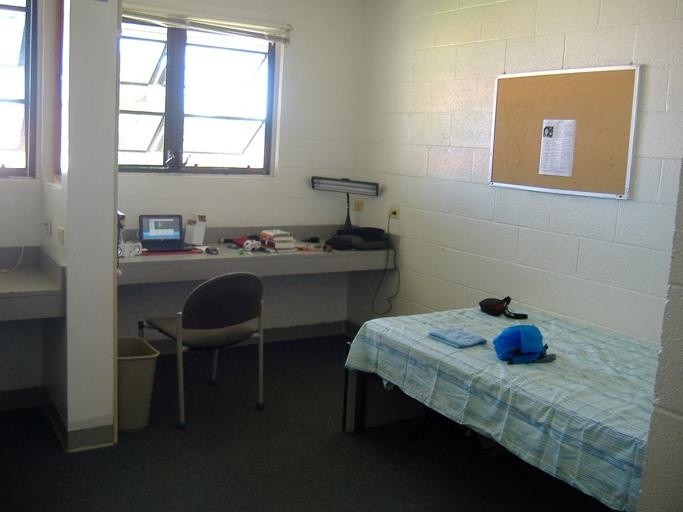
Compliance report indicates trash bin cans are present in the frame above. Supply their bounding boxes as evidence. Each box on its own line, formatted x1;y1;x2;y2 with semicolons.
117;335;160;431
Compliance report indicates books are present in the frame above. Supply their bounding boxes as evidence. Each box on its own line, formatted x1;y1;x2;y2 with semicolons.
258;229;302;256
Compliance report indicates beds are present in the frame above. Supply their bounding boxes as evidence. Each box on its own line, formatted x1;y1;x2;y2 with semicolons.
341;300;660;512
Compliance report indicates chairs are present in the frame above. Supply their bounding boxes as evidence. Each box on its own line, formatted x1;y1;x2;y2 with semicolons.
136;271;265;432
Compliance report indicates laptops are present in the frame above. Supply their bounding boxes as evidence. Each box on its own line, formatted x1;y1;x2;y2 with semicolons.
138;214;193;252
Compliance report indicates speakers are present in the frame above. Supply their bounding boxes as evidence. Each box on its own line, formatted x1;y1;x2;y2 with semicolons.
118;242;142;258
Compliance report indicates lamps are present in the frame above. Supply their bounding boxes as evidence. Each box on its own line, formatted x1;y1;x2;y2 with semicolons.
310;175;378;235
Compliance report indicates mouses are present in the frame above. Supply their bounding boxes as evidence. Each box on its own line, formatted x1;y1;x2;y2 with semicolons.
205;246;219;255
